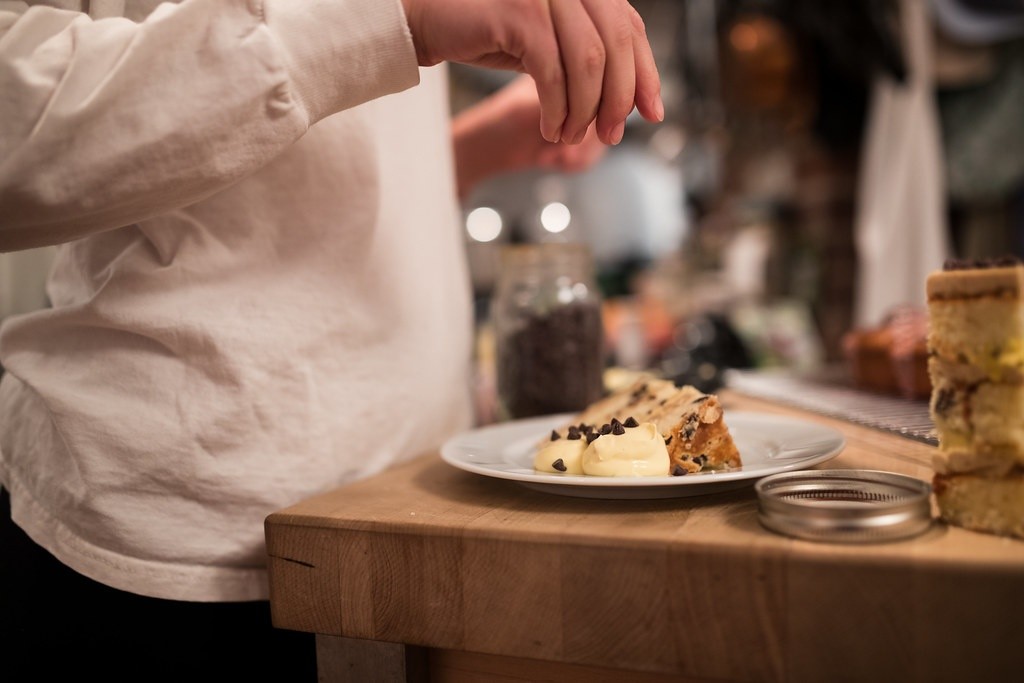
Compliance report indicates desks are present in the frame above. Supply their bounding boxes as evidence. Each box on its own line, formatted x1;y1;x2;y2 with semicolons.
263;365;1024;683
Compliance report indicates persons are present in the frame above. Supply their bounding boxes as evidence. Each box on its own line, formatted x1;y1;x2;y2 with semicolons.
0;0;666;683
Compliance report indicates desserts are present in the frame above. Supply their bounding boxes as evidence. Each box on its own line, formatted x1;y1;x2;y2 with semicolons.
925;255;1024;541
534;376;743;478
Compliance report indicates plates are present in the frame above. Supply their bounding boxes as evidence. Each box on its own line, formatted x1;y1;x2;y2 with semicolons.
439;412;847;501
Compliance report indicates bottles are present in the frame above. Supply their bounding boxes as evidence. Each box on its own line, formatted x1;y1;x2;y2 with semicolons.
488;243;604;421
